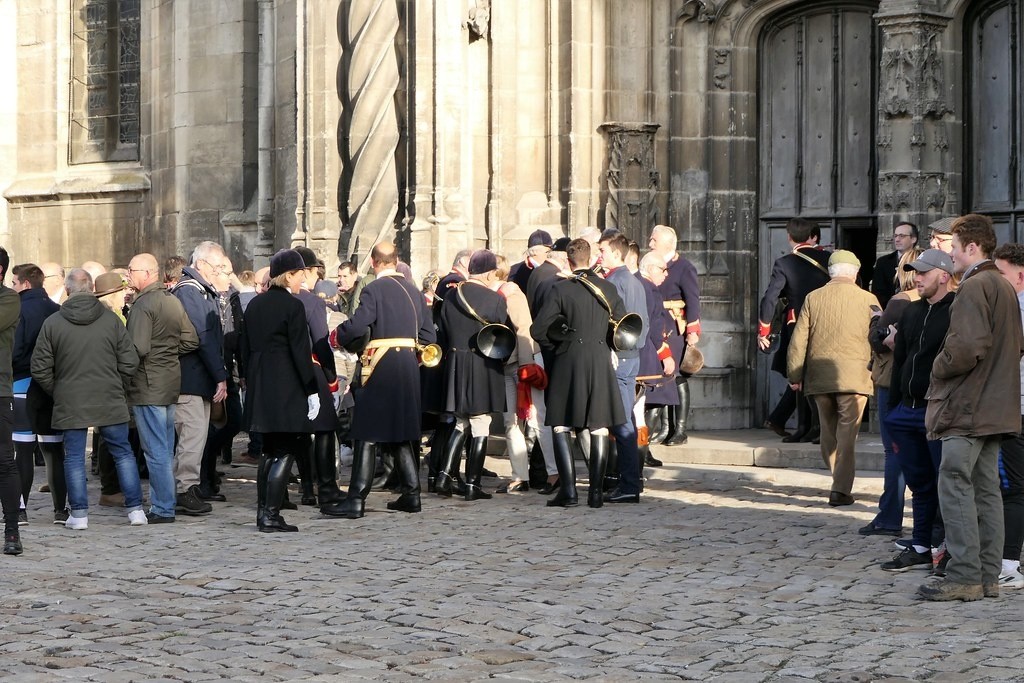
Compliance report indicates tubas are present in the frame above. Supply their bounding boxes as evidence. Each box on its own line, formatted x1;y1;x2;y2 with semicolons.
609;313;644;352
477;323;519;360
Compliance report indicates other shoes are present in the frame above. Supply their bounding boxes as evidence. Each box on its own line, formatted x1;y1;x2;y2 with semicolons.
831;489;1024;602
0;430;255;555
764;417;820;445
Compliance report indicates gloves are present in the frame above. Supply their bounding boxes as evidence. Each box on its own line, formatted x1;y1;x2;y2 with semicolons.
331;390;340;410
307;393;320;419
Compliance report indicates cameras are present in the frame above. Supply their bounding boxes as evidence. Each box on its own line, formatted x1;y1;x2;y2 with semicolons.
876;327;891;341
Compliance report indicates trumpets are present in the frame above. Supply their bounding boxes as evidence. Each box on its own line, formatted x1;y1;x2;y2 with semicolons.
413;341;442;368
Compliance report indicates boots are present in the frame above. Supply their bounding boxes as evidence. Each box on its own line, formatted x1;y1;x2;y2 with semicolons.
256;381;691;533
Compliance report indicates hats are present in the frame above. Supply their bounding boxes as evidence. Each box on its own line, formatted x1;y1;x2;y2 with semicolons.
315;279;337;301
270;248;310;278
396;262;412;281
468;250;500;274
929;215;960;233
830;249;861;268
901;247;956;277
292;246;323;267
94;272;128;297
529;229;552;248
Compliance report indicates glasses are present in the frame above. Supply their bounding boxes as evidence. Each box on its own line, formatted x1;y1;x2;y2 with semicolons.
892;231;909;238
203;260;225;273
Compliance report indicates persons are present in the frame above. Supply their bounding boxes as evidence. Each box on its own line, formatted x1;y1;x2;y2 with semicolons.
860;217;964;576
993;243;1024;588
787;250;883;505
30;267;149;528
579;225;699;503
231;246;376;532
529;238;625;507
490;230;572;496
434;251;507;501
94;241;244;522
757;220;833;442
372;251;465;494
0;247;104;553
320;243;437;517
919;214;1024;601
